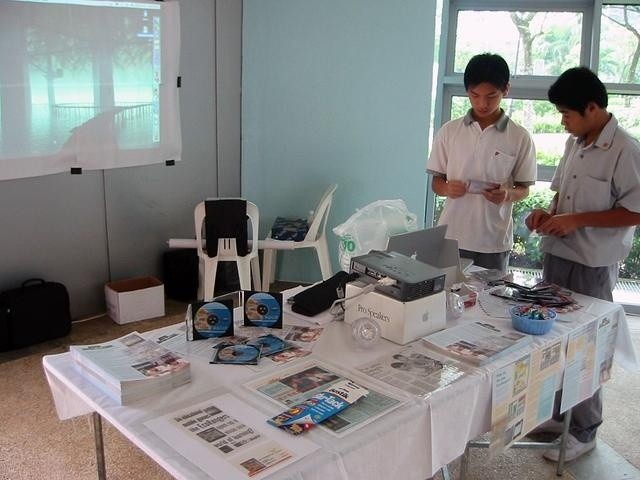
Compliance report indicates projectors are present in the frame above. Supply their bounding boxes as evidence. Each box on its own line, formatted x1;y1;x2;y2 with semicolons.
349;249;447;303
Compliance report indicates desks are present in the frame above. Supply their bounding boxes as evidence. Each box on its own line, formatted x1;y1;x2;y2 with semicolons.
43;264;624;480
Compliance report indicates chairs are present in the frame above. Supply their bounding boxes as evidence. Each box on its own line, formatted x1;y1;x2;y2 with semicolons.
195;184;342;302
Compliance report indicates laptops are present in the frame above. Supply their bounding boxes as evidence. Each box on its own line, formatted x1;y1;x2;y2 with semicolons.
438;239;474;272
385;224;448;266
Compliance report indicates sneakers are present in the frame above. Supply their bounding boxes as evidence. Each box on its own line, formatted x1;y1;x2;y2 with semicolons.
543;432;596;463
530;418;565;434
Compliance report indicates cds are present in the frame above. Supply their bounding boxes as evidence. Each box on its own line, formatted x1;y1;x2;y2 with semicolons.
194;301;232;338
248;336;285;353
244;293;281;328
218;344;260;362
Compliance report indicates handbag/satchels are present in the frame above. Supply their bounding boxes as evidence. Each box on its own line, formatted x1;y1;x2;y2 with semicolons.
1;279;71;353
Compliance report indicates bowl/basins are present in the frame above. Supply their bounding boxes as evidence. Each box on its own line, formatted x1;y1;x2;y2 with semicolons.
352;317;380;348
447;292;465;320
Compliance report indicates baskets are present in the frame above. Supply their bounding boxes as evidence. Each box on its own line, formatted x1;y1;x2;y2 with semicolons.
509;304;558;335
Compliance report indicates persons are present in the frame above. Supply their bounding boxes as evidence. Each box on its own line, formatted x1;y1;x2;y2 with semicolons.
525;64;640;465
144;365;169;378
270;327;333;393
164;357;188;372
425;50;538;271
393;354;443;373
391;362;431;378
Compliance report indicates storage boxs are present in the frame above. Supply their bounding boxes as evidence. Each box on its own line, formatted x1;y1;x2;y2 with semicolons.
104;274;165;326
